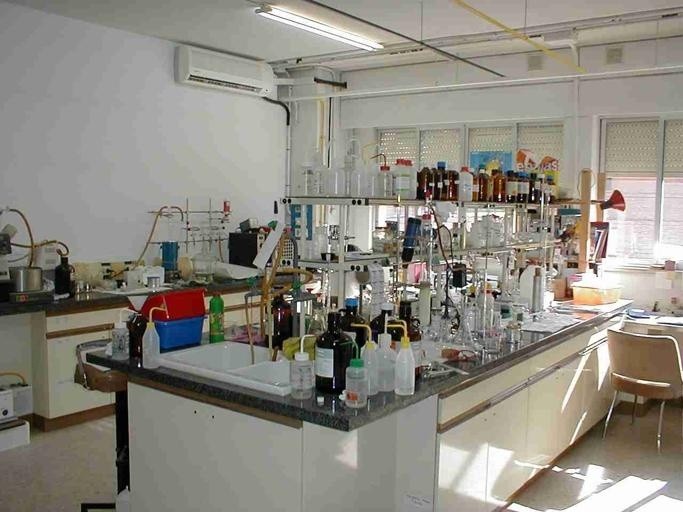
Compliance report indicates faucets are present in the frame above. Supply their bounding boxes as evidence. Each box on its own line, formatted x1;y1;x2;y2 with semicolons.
242;274;260;300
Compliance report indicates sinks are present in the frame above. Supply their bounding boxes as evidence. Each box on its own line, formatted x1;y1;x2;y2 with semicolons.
160;339;272;373
232;355;293;398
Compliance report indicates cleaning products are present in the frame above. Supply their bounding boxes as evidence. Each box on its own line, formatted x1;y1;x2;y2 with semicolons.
209;291;224;341
393;159;557;201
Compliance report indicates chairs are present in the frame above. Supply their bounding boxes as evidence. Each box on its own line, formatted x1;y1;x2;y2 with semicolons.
601;327;682;456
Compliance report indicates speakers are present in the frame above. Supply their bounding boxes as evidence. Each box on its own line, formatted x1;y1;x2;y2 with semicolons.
229;232;271;270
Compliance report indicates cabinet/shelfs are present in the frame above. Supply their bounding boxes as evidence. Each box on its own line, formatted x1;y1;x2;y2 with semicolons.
30;282;262;431
283;193;562;340
431;312;626;511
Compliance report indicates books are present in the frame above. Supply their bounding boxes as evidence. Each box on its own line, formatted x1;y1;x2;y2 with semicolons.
656;314;682;326
627;307;649;318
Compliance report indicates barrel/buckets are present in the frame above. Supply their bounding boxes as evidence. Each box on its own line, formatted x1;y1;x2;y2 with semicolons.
520;259;546;309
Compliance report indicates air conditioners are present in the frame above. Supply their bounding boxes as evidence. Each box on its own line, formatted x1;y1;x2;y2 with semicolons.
173;45;271;99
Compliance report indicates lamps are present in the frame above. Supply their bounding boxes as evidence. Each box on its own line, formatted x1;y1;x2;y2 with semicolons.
255;5;384;52
591;189;625;212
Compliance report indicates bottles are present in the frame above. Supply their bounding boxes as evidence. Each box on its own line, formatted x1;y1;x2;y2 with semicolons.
108;304;171;370
208;290;226;344
264;153;562;414
52;257;76;298
